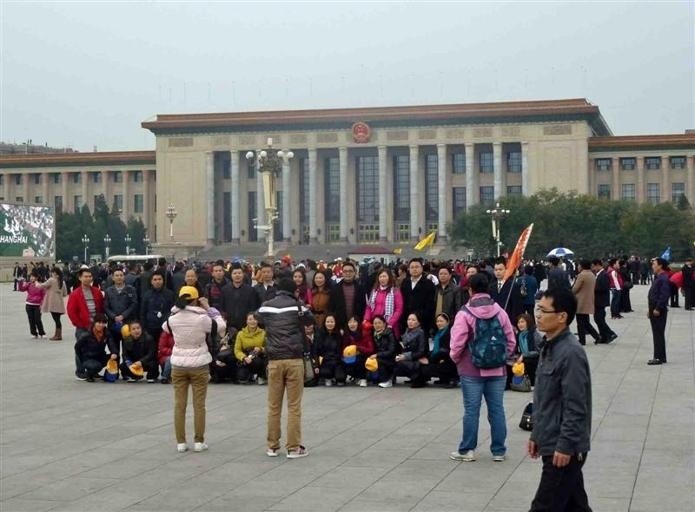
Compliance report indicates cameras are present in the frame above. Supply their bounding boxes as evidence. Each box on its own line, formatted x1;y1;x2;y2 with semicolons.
17;277;24;282
198;298;200;306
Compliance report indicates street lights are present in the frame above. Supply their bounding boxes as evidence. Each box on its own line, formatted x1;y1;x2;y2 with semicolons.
245;138;294;256
143;235;150;255
124;233;132;256
82;234;90;263
166;204;177;242
103;234;111;258
486;203;511;257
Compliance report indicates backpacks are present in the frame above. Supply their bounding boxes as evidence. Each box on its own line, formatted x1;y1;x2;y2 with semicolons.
460;305;507;368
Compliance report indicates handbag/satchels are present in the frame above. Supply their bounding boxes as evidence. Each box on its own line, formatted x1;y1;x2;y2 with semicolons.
520;284;528;297
624;280;632;289
509;374;531;391
520;404;533;431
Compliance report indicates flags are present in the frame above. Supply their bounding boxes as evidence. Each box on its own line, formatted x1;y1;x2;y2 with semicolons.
414;232;435;251
660;246;673;263
502;222;535;282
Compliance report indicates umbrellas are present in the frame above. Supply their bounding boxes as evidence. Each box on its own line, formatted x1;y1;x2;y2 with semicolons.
545;246;575;259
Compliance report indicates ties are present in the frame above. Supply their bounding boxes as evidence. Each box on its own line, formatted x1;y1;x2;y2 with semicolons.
498;282;502;293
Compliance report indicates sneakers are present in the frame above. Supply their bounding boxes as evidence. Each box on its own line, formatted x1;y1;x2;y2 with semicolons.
178;442;188;451
75;369;103;382
31;334;47;338
267;448;280;457
306;377;463;388
492;455;505;461
595;333;617;343
194;442;209;452
648;358;666;365
239;373;267;385
127;378;169;384
449;449;476;462
288;446;309;458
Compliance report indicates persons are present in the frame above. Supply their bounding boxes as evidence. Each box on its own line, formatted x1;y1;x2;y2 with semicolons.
13;255;695;391
161;286;226;453
527;287;592;512
253;277;315;458
449;273;516;461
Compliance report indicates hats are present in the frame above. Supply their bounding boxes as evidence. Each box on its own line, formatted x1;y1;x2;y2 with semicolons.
125;359;145;376
342;345;358;363
513;363;524;380
179;286;199;300
105;358;118;382
279;256;290;265
366;356;380;379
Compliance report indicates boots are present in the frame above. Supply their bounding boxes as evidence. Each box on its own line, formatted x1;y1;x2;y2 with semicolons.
49;328;62;341
